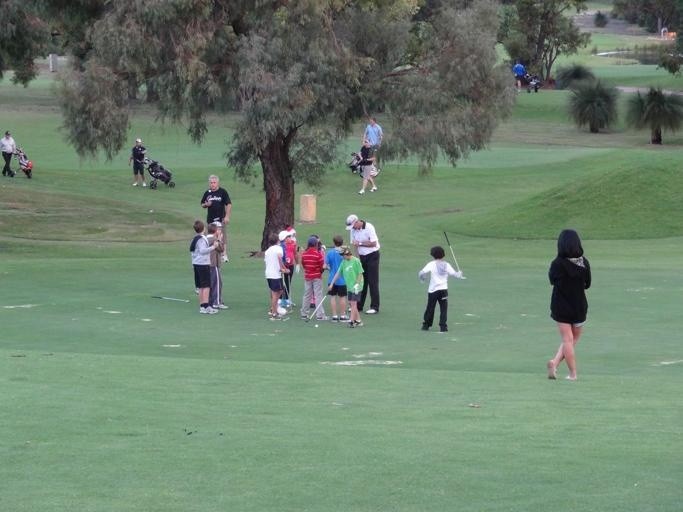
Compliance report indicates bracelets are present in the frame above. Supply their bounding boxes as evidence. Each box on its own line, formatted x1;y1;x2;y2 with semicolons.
357;242;362;246
204;204;206;207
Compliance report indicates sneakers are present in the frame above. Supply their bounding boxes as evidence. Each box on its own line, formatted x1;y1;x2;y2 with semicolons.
142;182;147;187
2;171;6;176
364;308;380;314
10;171;16;177
331;315;363;328
132;182;139;187
200;302;229;314
358;189;365;194
268;297;296;320
369;187;378;193
565;374;578;381
440;325;449;332
348;308;353;312
421;323;429;330
301;313;312;320
222;254;229;263
547;360;557;380
316;314;329;321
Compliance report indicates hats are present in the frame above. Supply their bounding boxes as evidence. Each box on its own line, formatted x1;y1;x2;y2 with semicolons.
336;246;353;255
345;214;359;231
278;225;297;242
307;237;320;245
136;139;142;143
212;221;223;227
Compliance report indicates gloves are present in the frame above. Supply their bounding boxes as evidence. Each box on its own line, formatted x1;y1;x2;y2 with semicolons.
296;263;303;273
352;284;360;295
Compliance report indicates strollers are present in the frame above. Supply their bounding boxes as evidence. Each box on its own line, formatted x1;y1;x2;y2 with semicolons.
128;154;176;189
347;141;370;177
524;74;543;94
8;148;38;180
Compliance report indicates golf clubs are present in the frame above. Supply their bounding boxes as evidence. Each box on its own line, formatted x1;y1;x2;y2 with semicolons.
444;232;467;280
305;292;330;322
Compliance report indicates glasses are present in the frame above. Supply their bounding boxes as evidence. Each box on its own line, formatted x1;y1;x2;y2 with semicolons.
8;134;11;135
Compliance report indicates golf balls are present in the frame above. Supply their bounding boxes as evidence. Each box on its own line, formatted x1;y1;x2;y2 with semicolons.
314;325;319;328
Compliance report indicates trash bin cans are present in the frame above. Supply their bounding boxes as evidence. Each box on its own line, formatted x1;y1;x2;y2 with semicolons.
300;194;317;224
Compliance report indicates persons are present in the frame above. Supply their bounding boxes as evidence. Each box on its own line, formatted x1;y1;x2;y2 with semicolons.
345;215;381;315
260;225;365;328
362;118;384;167
358;138;377;193
189;219;218;313
526;74;543;87
418;246;464;331
545;227;590;382
512;58;525;90
204;223;228;309
201;175;232;262
0;131;16;178
127;138;147;188
